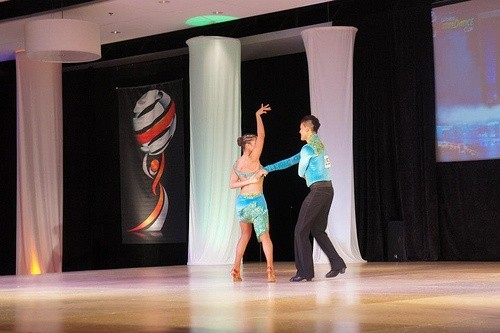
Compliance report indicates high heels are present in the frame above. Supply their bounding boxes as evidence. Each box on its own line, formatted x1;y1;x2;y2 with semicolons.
325;266;345;278
231;269;242;282
266;267;276;282
290;274;314;281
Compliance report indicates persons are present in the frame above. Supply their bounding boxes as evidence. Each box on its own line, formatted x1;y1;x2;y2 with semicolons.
229;104;276;282
254;115;346;282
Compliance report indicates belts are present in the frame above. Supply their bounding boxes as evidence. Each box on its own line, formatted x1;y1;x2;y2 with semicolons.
310;181;332;190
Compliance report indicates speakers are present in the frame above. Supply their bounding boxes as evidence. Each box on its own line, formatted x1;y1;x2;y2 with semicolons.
385;220;408;262
